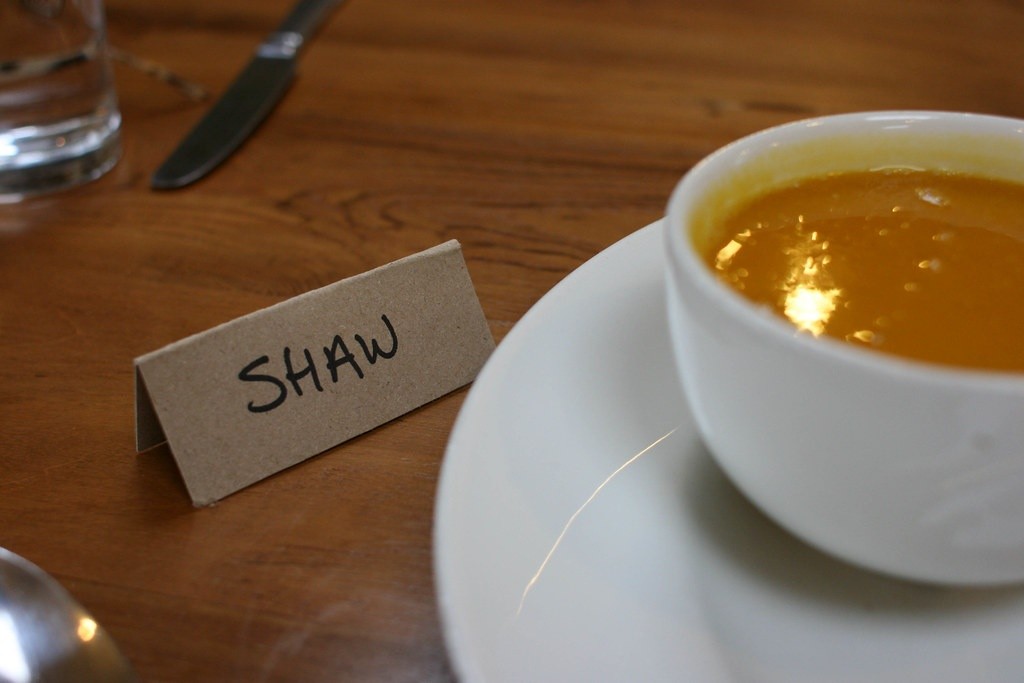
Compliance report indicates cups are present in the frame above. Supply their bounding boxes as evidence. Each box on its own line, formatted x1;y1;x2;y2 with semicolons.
1;1;123;204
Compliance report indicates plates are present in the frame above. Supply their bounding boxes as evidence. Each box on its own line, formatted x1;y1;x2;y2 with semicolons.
432;216;1023;682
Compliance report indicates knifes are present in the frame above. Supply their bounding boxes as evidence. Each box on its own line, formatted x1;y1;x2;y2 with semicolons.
150;0;340;187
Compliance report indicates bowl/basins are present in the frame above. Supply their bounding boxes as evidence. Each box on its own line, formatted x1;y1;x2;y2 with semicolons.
663;111;1024;585
